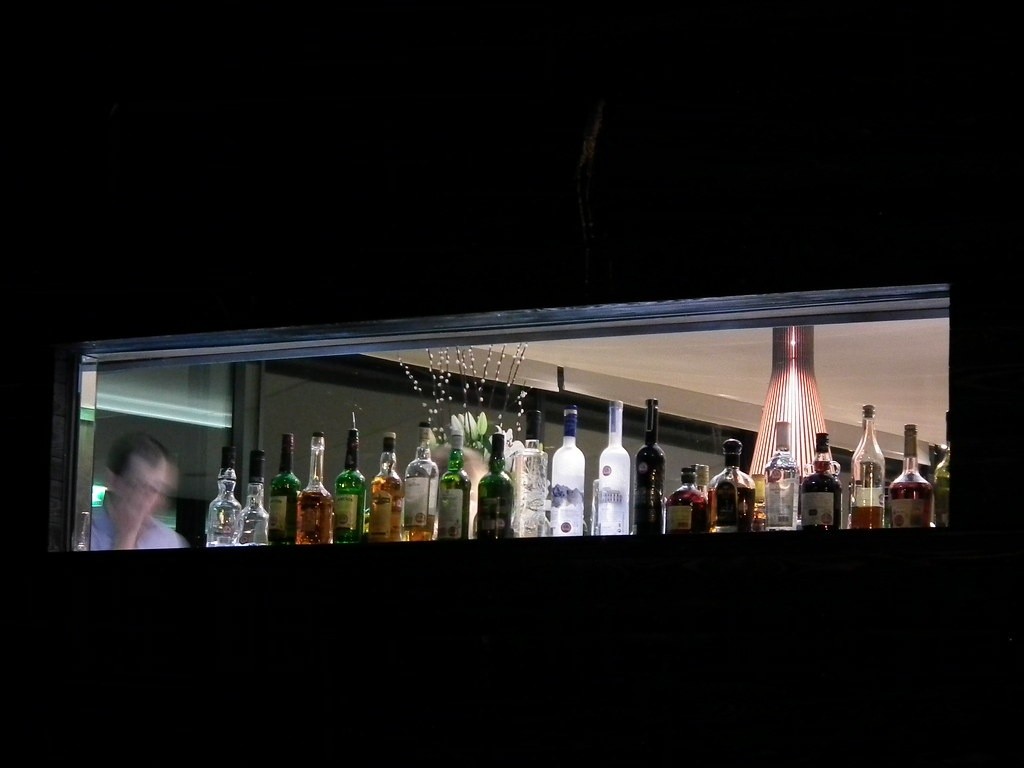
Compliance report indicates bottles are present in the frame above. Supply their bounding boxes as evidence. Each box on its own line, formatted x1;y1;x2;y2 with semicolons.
235;449;267;544
515;410;548;537
296;432;333;542
799;404;949;529
403;422;437;540
333;428;367;542
265;433;302;543
597;398;757;530
437;435;472;541
765;422;800;530
205;446;242;546
368;432;403;540
475;433;511;538
550;404;585;537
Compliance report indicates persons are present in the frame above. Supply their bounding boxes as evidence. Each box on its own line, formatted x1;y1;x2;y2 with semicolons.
92;431;191;550
429;445;491;539
